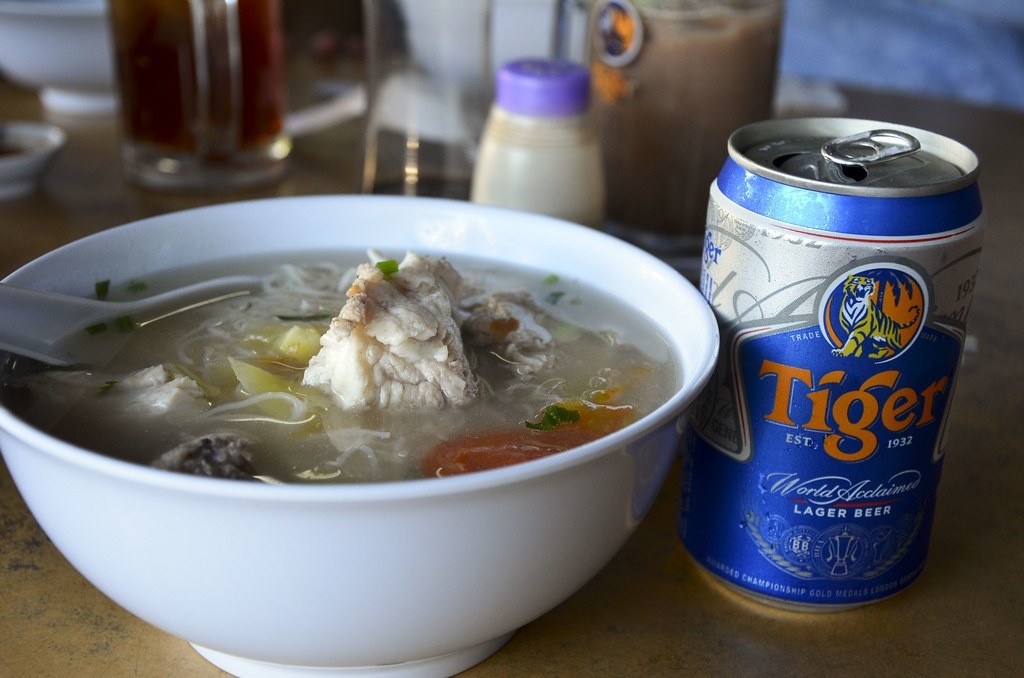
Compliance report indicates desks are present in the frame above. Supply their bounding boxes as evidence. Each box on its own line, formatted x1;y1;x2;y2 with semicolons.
0;89;1024;678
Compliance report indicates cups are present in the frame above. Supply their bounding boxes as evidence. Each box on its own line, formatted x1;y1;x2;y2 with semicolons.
579;0;785;275
362;0;493;202
101;1;292;186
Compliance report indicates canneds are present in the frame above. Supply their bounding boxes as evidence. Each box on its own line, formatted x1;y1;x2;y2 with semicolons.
678;115;985;614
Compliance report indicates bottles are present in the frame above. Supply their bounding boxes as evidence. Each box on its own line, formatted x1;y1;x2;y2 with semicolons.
466;57;607;232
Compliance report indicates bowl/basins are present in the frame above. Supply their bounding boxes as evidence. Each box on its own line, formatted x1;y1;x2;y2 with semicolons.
0;1;116;115
0;120;68;200
0;194;719;678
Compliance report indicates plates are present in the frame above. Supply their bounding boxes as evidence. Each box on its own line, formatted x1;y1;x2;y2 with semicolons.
286;80;366;138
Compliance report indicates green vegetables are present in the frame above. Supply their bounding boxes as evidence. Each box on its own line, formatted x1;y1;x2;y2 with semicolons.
41;258;581;432
